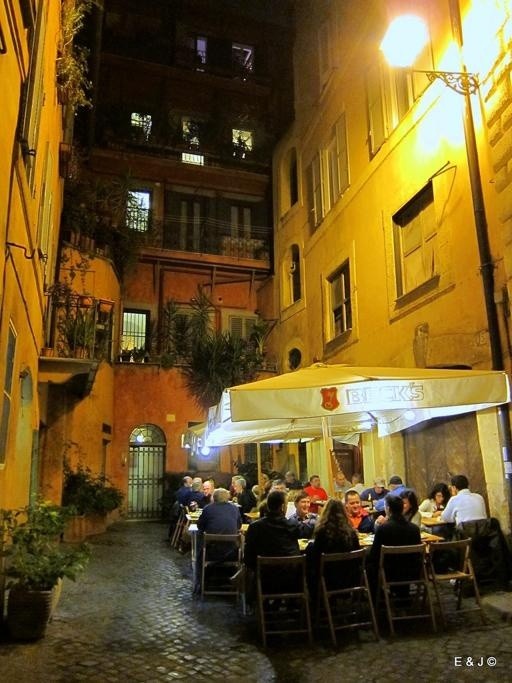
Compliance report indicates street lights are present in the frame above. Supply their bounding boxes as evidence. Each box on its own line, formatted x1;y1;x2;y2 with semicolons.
373;9;511;494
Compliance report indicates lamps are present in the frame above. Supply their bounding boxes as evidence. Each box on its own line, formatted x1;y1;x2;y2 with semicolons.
136;428;148;443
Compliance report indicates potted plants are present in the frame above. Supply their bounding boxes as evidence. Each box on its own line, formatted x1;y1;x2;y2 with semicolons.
60;455;127;544
113;295;280;371
60;163;138;257
0;495;92;640
40;284;117;364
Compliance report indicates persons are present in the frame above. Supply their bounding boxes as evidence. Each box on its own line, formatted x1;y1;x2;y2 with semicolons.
173;470;494;613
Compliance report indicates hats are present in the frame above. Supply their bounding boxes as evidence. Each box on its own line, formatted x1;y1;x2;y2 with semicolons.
388;475;401;485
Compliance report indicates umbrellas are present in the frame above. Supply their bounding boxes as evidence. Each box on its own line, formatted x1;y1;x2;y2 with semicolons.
203;357;510;497
204;403;371;488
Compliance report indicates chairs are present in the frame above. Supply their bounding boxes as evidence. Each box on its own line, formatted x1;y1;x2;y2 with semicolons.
168;495;512;654
